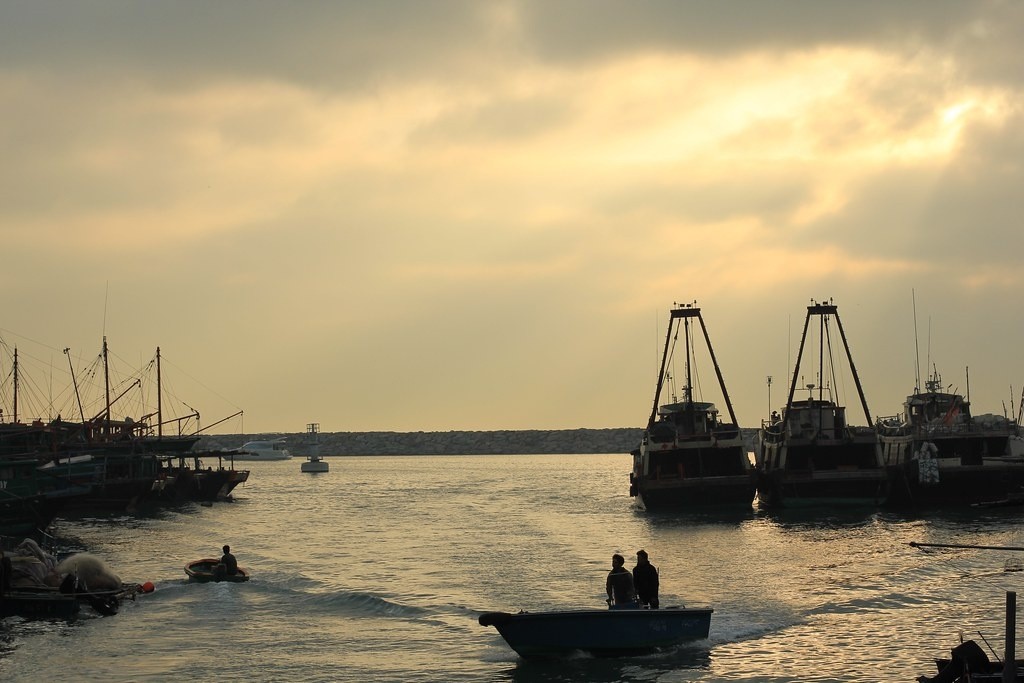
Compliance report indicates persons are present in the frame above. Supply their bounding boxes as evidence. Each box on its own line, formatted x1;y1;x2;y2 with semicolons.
633;550;659;611
218;545;238;576
606;553;634;605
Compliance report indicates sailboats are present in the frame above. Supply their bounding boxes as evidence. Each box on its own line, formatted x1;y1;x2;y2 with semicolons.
0;336;251;536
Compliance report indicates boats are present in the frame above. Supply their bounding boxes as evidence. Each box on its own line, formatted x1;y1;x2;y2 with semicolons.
478;606;714;660
755;299;894;516
223;439;293;461
184;558;249;583
877;287;1023;514
0;542;143;617
628;298;760;514
